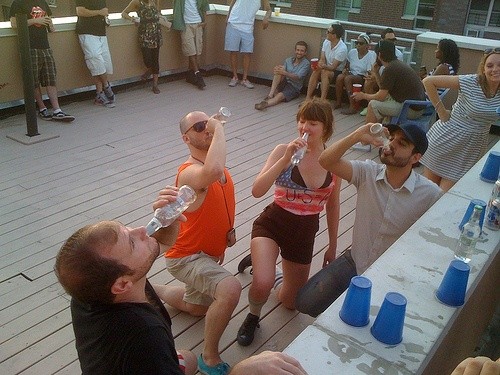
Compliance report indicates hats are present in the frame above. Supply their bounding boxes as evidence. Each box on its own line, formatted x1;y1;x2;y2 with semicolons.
385;123;428;154
359;34;370;44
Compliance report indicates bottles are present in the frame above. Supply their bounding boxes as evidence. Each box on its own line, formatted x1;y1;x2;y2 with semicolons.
214;107;231;126
454;205;483;263
291;133;309;165
370;123;390;152
145;185;197;237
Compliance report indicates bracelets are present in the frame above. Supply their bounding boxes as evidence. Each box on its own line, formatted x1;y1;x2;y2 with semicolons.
204;21;207;26
131;16;135;23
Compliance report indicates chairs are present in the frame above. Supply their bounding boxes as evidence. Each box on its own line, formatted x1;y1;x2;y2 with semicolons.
390;88;450;134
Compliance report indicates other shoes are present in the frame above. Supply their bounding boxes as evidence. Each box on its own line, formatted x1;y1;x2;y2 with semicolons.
229;79;239;86
190;72;205;87
153;87;160;94
241;79;254;88
255;101;267;110
334;104;343;110
264;95;275;101
143;71;151;81
341;109;357;114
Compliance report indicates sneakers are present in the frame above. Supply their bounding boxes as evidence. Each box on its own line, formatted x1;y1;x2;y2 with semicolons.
198;352;230;375
95;97;115;108
104;82;115;100
52;111;75;121
237;313;260;346
360;107;367;115
238;254;252;273
39;109;52;120
351;142;371;152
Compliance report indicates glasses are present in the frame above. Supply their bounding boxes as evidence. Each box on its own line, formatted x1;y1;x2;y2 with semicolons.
387;38;396;42
184;120;208;134
485;48;500;54
327;31;331;34
355;41;367;45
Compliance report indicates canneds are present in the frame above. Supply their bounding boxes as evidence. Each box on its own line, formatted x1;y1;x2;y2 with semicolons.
483;199;500;230
488;179;500;205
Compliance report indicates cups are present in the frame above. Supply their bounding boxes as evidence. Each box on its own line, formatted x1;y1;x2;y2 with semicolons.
353;84;362;95
274;8;280;16
436;260;470;306
370;292;407;345
311;59;318;69
459;199;487;235
339;276;372;327
480;151;500;183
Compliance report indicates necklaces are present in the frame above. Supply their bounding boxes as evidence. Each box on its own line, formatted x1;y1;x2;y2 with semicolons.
191;154;237;247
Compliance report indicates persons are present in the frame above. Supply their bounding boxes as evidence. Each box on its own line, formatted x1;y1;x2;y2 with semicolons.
449;356;500;375
121;0;163;94
254;41;312;110
8;0;75;122
295;122;444;318
74;0;116;108
298;22;460;152
418;48;500;194
172;0;210;88
53;220;310;375
149;111;242;375
237;96;343;347
224;0;272;89
